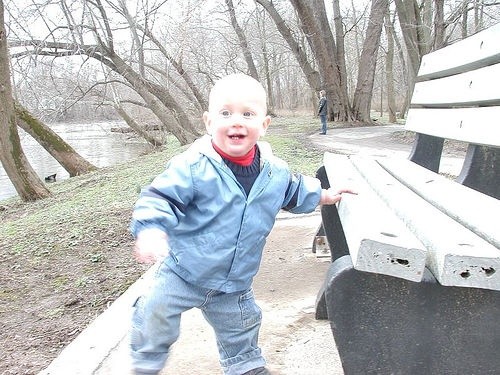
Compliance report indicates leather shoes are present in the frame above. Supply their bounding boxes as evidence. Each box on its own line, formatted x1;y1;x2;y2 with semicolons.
320;133;326;135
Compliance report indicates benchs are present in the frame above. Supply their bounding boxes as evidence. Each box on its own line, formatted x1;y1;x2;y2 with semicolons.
312;20;500;374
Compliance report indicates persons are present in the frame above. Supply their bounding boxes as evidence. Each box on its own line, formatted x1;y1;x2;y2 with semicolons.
131;74;359;375
317;89;329;135
